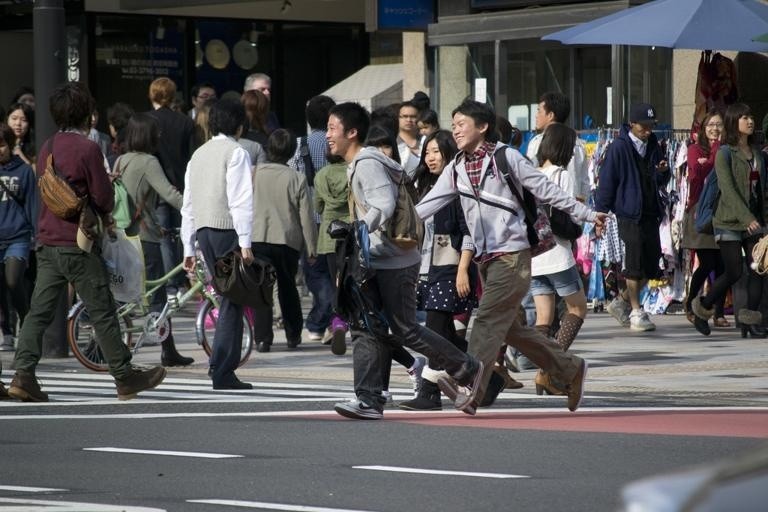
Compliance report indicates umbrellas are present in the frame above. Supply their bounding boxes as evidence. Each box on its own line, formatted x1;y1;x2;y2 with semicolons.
540;0;768;54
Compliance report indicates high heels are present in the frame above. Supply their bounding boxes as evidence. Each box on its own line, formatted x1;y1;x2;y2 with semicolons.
737;309;768;338
533;367;569;398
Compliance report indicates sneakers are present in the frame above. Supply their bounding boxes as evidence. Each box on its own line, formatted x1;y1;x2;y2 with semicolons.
437;360;484;417
257;316;350;357
606;294;657;332
334;396;383;420
491;364;523;390
568;354;588;411
410;355;426;396
380;389;394;409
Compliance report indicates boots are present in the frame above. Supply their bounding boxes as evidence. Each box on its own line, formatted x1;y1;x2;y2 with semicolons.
110;367;167;402
158;320;195;366
8;368;52;404
396;366;443;411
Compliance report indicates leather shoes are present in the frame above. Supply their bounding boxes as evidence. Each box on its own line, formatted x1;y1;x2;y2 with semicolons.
213;377;252;390
207;361;220;376
691;295;716;336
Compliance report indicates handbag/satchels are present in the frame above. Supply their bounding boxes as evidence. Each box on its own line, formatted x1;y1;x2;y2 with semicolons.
98;226;148;305
550;204;584;240
212;254;276;309
37;165;82;220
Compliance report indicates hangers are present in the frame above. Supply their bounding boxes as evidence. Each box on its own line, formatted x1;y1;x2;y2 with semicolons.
596;127;692;143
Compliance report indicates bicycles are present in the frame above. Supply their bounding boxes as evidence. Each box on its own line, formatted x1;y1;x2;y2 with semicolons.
65;227;254;375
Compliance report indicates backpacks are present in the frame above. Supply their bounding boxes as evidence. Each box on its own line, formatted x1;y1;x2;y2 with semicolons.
452;146;556;259
345;153;425;256
110;150;158;228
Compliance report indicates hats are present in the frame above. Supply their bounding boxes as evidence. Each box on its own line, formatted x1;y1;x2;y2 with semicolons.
629;101;658;127
